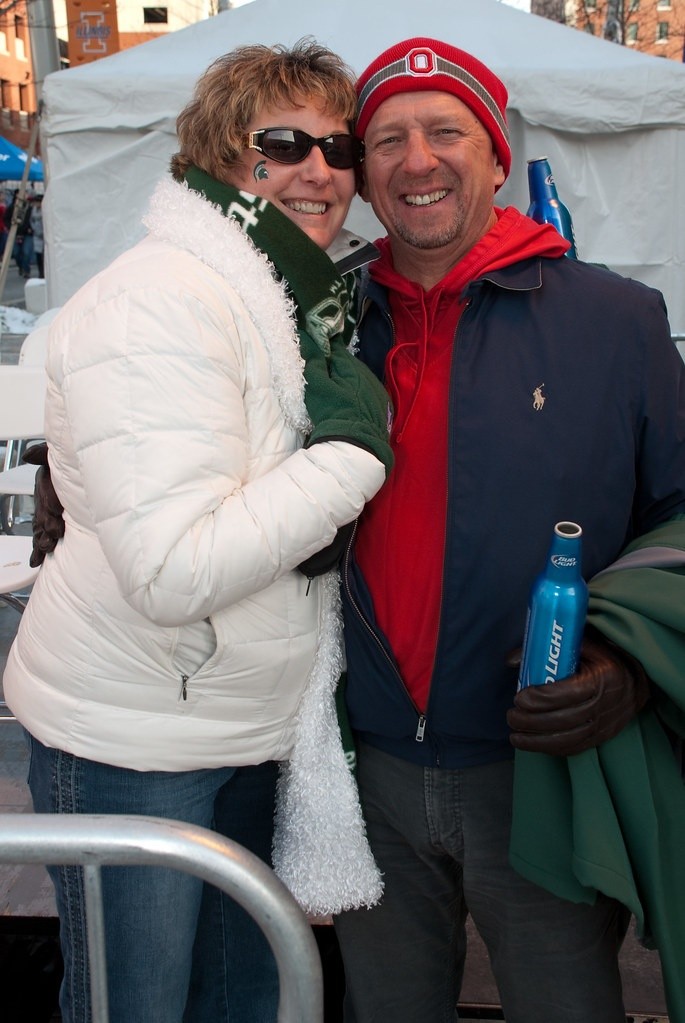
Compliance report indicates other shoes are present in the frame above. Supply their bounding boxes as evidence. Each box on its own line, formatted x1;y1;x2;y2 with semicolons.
18;267;23;276
24;271;31;279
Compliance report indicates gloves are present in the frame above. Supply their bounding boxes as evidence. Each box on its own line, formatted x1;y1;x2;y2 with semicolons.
507;637;655;754
295;329;394;476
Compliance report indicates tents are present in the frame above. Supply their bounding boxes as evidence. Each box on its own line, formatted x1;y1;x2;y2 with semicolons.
0;135;42;182
42;0;685;360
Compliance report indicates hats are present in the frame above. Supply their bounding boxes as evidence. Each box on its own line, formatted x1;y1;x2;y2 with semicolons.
349;37;512;194
33;194;43;202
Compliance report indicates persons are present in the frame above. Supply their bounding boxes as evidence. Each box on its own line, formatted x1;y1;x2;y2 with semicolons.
0;188;44;278
331;36;685;1023
4;35;396;1023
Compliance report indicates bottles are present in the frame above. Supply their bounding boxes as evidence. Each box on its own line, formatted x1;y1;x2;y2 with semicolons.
516;520;586;693
525;156;577;261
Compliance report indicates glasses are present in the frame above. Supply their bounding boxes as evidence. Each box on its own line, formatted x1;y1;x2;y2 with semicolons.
240;126;366;170
